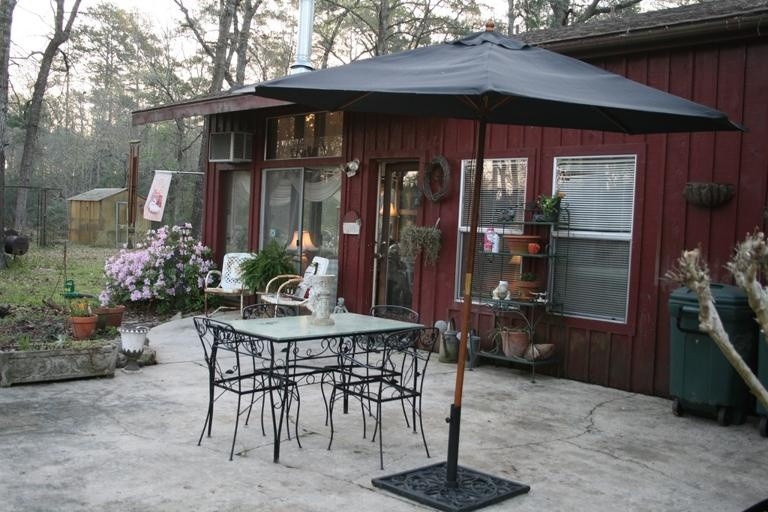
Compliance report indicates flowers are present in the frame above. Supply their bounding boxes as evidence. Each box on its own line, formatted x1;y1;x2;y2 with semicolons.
536;188;566;217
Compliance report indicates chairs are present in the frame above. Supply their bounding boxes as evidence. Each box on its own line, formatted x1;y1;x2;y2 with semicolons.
204;252;329;318
192;317;302;460
241;304;418;438
328;326;438;469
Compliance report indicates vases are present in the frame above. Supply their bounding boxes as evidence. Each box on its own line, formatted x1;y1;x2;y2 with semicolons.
502;233;542;254
117;325;148;355
544;213;557;221
536;188;566;217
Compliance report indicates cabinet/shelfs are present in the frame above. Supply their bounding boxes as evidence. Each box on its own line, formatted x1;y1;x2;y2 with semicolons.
468;206;569;384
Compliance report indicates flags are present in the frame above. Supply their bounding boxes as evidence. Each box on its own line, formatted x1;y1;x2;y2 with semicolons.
502;233;542;254
117;325;148;355
544;213;557;221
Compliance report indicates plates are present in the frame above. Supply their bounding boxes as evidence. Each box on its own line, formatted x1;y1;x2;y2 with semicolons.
468;206;569;384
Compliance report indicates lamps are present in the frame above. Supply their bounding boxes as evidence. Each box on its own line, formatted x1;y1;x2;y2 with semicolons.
204;252;329;318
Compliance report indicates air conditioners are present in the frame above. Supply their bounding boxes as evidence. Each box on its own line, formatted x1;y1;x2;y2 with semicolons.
208;132;251;163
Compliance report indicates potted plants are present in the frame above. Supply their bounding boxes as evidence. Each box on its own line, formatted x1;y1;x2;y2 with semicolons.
514;271;542;298
69;296;126;339
488;325;536;359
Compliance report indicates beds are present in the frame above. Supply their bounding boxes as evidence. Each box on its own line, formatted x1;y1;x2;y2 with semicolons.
232;17;748;482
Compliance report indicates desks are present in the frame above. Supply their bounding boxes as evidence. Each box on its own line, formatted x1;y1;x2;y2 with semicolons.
196;313;424;462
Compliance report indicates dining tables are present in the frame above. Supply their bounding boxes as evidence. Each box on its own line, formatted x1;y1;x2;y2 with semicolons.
69;296;126;339
488;325;536;359
514;271;542;298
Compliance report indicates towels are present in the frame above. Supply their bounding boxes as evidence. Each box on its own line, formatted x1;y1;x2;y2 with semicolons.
668;281;768;437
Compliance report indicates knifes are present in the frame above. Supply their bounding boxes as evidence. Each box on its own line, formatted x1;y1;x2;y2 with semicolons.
208;132;251;163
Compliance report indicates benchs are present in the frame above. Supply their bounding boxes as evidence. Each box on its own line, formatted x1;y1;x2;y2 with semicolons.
144;171;174;223
